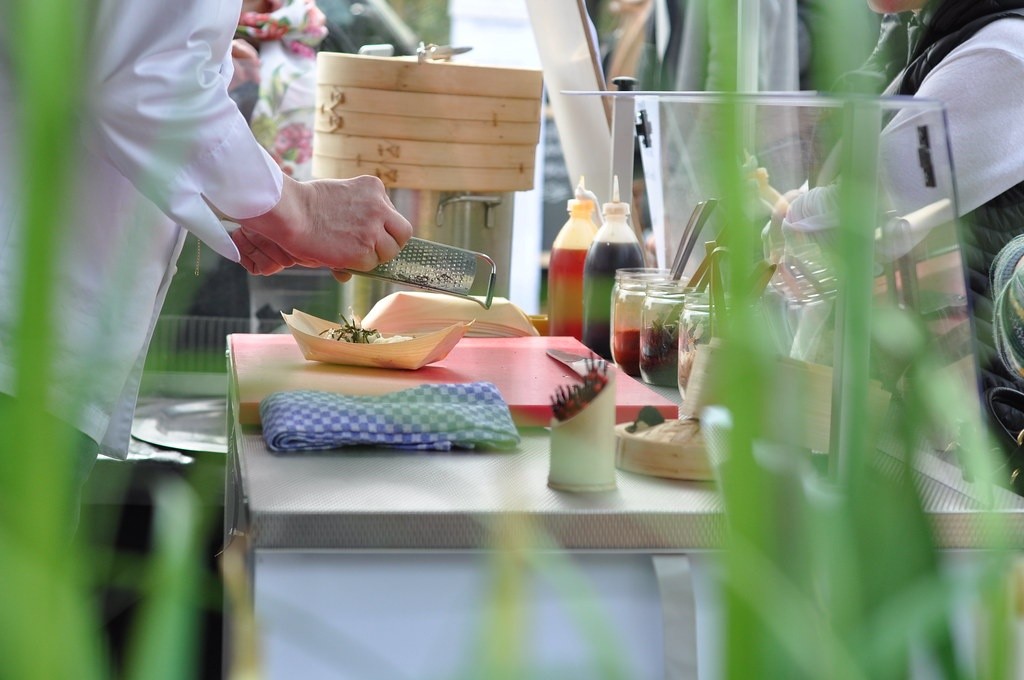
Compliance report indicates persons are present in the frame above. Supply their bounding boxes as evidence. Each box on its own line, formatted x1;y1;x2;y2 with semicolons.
601;0;1024;496
0;0;414;569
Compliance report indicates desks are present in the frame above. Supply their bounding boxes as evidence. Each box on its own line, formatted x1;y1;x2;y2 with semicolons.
221;330;733;679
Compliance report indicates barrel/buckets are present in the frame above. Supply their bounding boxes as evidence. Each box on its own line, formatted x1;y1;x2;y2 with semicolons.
344;185;514;342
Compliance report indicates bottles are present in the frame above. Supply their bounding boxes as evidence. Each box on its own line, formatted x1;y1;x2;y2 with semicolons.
547;176;602;342
583;175;645;362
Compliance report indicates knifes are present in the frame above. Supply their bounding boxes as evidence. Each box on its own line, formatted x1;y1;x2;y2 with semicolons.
546;349;605;383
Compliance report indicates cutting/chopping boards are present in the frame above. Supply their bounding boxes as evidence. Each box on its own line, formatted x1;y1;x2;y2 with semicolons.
231;333;679;427
